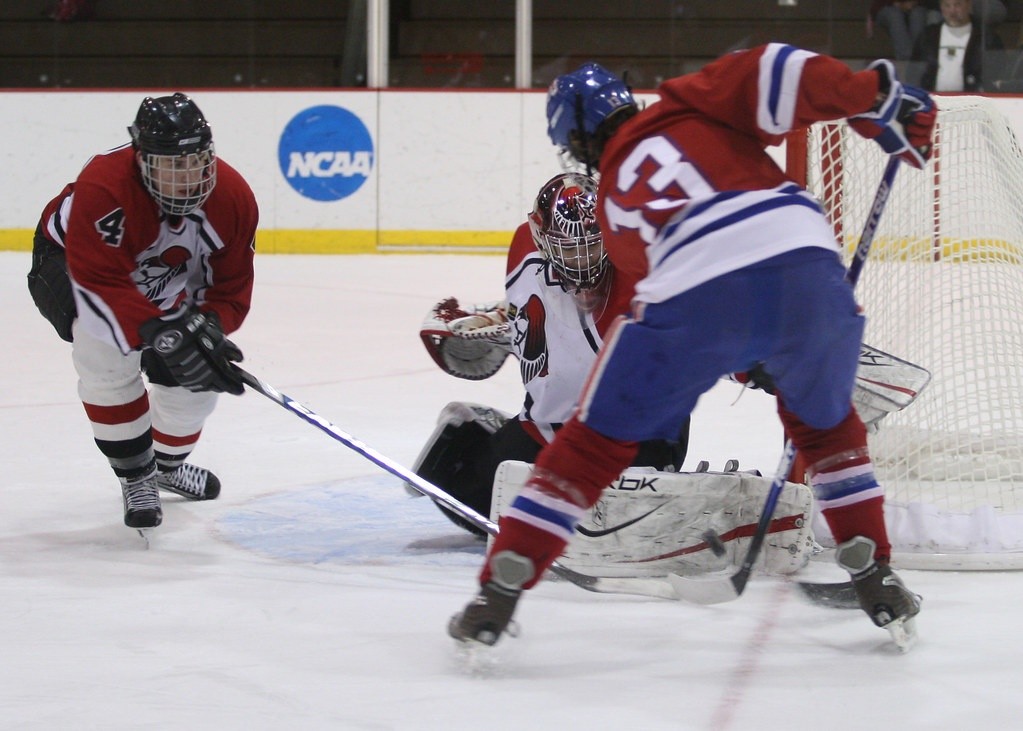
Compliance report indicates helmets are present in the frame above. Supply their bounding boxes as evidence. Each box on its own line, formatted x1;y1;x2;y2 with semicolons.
127;91;219;214
547;62;640;159
527;173;612;290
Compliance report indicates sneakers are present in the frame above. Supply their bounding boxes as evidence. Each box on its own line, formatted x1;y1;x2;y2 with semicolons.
152;463;222;500
450;577;522;668
835;537;923;650
119;471;163;541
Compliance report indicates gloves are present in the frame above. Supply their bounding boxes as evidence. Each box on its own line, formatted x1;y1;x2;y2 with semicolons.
845;54;940;171
137;304;247;396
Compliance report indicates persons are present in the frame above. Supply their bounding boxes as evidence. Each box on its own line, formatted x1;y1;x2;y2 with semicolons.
406;174;691;542
869;1;927;61
26;93;260;528
449;42;938;647
906;0;1003;92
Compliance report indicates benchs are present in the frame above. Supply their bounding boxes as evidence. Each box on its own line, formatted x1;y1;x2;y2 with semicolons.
2;0;1022;89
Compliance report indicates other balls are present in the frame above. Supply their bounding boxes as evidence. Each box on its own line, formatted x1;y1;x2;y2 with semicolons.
701;528;729;560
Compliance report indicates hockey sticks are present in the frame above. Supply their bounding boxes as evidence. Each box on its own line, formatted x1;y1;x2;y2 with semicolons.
667;154;901;606
227;360;682;601
